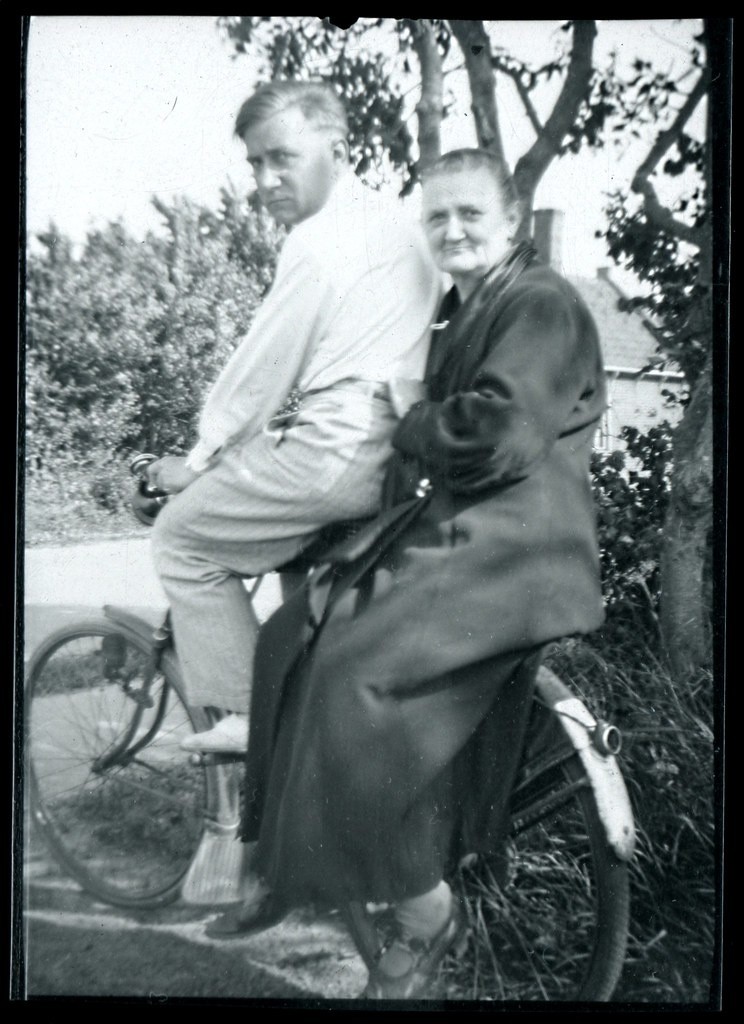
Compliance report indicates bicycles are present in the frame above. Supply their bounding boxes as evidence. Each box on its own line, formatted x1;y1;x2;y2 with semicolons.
21;443;636;1003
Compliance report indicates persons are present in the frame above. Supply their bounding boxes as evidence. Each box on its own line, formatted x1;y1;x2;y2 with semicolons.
145;79;439;755
202;149;606;998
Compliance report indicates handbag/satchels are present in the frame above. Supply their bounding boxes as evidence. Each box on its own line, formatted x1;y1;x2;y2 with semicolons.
316;479;436;587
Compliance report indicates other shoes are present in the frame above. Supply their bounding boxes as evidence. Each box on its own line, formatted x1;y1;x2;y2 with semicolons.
357;896;463;999
180;714;250;756
207;881;307;937
